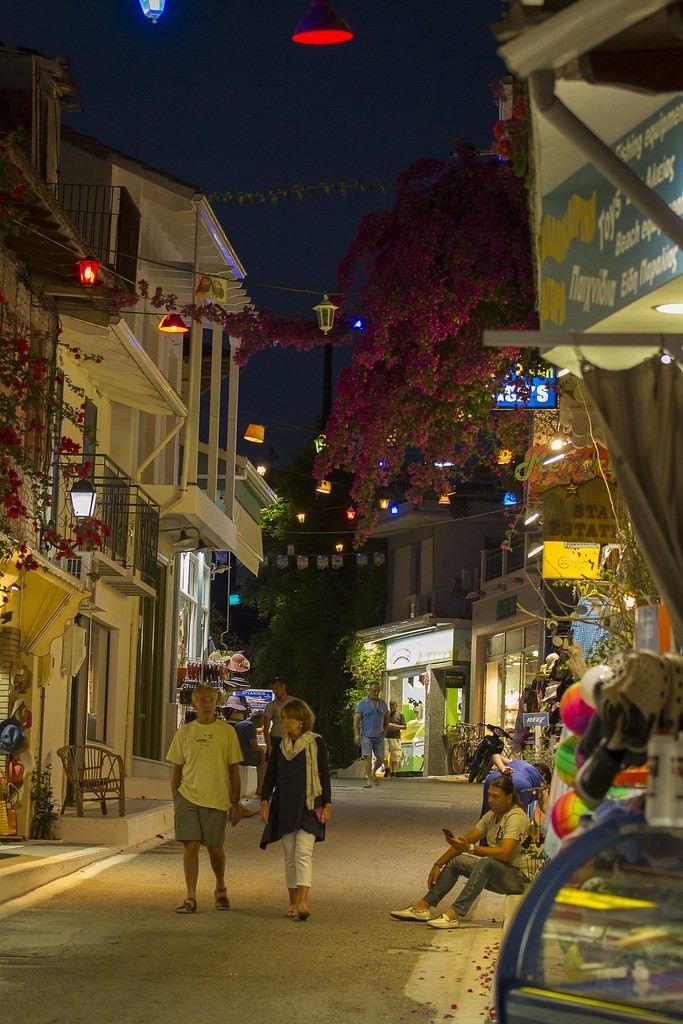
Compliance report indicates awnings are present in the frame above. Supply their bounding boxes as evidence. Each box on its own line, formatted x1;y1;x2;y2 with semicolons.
6;534;95;659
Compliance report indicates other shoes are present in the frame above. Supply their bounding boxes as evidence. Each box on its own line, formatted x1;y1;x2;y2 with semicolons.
363;784;372;788
391;772;399;777
298;907;310;919
284;906;298;917
383;768;391;778
373;780;380;786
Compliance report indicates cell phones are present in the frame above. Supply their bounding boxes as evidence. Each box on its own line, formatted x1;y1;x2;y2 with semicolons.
442;828;455;838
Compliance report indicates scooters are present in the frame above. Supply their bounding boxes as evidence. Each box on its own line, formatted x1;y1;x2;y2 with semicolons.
467;723;513;783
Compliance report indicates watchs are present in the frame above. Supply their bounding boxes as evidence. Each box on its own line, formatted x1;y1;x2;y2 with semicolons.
468;844;474;854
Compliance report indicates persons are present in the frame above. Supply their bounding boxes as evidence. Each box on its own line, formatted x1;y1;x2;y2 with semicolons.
353;682;389;787
383;699;407;778
264;679;314;767
233;710;275;798
477;753;551;850
390;775;532;928
260;699;332;921
165;685;243;914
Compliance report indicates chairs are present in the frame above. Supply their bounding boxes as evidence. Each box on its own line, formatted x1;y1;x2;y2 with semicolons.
56;743;125;818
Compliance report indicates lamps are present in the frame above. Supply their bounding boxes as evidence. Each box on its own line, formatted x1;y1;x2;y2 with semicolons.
71;248;104;289
310;292;341;336
527;541;544;559
155;516;196;546
255;462;268;477
542;449;565;466
520;513;542;526
181;545;235;574
314;435;330;453
39;468;98;554
296;510;308;524
438;493;452;505
244;423;264;444
347;505;357;521
155;311;192;335
335;539;344;554
159;525;215;554
316;480;332;494
290;0;355;47
503;491;518;506
380;496;389;510
497;446;516;465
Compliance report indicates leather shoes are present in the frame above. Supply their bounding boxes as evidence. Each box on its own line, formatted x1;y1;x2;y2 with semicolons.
390;905;431;921
426;913;460;928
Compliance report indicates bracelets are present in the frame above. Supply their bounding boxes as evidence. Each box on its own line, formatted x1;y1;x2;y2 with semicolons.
434;863;442;868
230;801;238;803
328;803;333;806
266;742;272;744
397;726;398;728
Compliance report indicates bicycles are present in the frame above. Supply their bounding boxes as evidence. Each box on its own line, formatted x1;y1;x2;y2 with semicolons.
442;722;486;775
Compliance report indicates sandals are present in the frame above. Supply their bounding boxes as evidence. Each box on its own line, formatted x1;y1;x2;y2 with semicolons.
214;887;230;909
177;898;198;914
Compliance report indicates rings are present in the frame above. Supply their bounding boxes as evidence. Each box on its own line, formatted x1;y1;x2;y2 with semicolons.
259;816;262;818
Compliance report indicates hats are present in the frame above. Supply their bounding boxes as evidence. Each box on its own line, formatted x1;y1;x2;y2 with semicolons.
219;696;247;710
533;653;561;713
0;660;32;794
226;654;250;672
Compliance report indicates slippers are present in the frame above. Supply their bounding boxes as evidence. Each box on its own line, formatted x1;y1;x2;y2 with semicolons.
596;649;681;753
